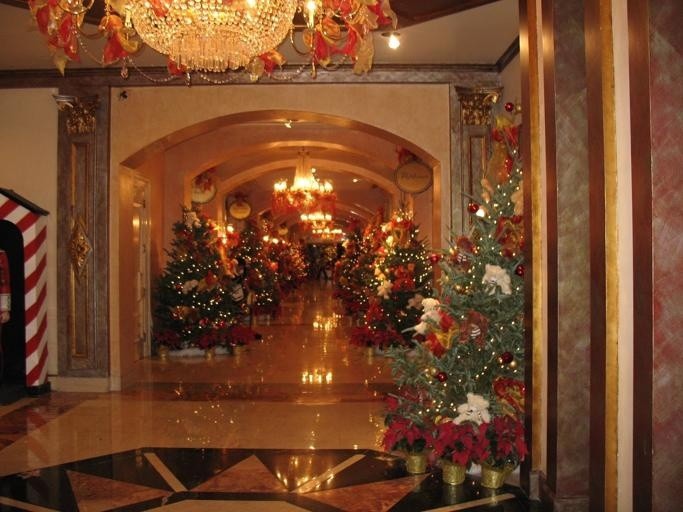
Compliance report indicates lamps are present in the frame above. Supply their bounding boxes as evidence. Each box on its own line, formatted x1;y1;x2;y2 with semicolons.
25;1;399;86
227;110;355;242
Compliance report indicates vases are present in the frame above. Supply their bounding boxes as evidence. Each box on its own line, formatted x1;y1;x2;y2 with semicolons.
406;455;506;495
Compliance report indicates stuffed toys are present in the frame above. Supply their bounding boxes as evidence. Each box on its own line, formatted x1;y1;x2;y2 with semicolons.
412;298;440;333
375;281;394;300
481;263;511;295
183;211;202;230
405;294;423;310
373;267;386;283
181;280;198;295
455;309;487;349
451;392;491;426
446;236;475;274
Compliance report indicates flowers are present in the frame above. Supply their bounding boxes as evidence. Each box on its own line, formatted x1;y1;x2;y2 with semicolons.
144;203;310;358
330;206;434;357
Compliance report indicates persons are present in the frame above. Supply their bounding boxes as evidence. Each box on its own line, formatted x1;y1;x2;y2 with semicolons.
314;253;329;281
